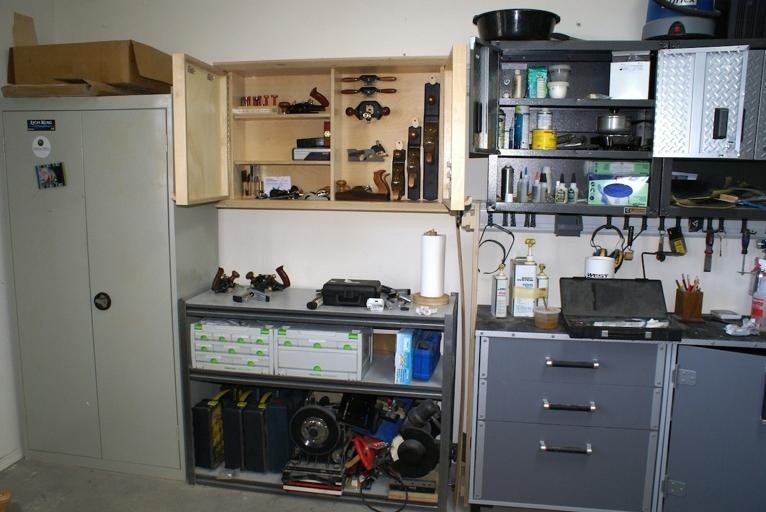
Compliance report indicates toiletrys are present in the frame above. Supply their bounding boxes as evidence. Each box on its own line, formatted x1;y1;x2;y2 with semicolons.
537;264;550;309
491;265;509;318
510;239;537;318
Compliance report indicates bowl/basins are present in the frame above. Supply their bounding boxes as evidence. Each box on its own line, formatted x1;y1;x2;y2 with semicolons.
474;9;560;40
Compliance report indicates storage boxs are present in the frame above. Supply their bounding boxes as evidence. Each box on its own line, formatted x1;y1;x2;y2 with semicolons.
7;39;173;94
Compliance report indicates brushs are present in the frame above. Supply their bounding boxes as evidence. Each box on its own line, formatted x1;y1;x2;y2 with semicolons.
624;226;634;260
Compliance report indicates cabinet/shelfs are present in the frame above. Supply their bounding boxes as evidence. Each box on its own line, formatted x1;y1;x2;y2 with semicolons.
0;93;219;482
487;40;670;218
660;38;766;221
173;43;467;213
178;284;459;512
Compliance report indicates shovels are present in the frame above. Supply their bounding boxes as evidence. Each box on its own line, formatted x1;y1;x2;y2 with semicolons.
704;226;714;272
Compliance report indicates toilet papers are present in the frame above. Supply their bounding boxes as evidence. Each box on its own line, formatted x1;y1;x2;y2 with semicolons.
420;235;446;297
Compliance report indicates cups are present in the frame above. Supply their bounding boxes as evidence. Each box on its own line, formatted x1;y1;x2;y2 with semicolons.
675;288;704;319
498;109;505;149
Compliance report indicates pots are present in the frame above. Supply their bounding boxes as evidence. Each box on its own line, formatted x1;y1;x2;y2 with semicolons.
596;108;655;134
597;184;633;205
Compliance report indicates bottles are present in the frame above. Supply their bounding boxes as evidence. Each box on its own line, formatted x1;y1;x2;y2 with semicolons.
509;106;529;149
512;69;522;99
537;108;553;130
537;76;546;98
501;162;579;205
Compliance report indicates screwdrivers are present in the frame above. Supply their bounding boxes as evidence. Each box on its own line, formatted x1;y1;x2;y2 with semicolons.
741;229;751;276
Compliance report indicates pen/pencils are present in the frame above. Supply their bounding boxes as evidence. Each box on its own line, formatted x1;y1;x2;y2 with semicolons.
676;273;701;293
